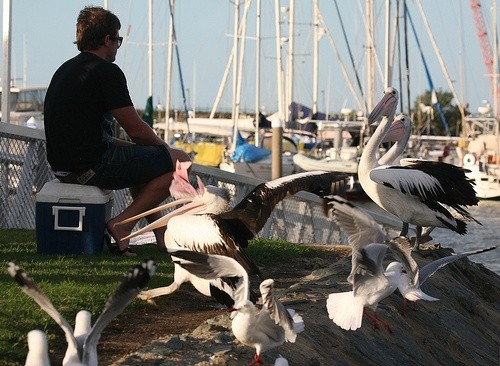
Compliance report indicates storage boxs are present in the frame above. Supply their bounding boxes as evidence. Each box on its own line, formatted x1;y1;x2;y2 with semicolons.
35;178;115;256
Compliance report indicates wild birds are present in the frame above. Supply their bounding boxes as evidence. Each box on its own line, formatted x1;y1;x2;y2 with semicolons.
358;86;480;248
320;194;480;335
114;171;350;312
167;250;305;366
6;256;158;366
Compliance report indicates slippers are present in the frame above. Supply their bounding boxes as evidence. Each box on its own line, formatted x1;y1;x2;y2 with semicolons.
104;234;131;256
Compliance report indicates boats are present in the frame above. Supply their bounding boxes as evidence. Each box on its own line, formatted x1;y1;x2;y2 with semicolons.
0;41;49;130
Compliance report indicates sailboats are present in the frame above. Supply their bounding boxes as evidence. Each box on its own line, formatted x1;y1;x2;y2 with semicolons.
102;0;500;200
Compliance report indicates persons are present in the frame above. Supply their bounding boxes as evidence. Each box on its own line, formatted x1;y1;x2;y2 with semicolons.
43;5;193;259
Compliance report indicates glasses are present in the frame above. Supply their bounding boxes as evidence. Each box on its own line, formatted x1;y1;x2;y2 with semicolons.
108;37;124;48
